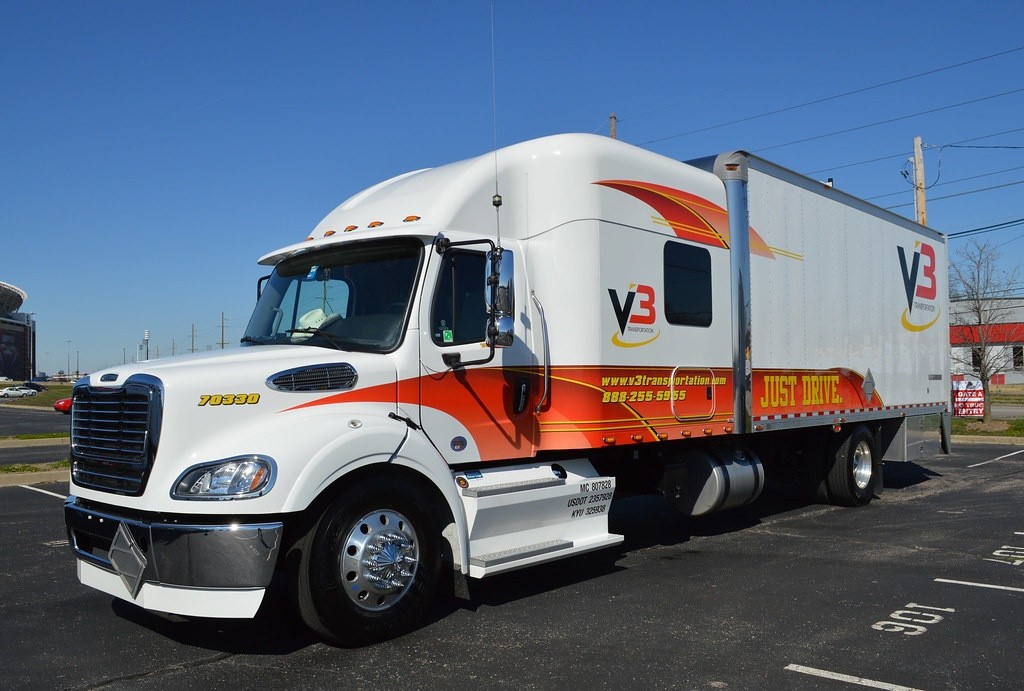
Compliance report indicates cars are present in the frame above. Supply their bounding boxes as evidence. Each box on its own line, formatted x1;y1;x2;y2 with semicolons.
53;398;72;415
0;382;48;398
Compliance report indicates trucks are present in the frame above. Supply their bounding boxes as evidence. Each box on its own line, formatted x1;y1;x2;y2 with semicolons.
64;132;951;648
0;377;11;382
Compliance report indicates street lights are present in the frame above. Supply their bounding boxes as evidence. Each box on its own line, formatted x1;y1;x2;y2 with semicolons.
145;330;149;360
66;340;72;381
123;348;125;364
76;350;79;378
